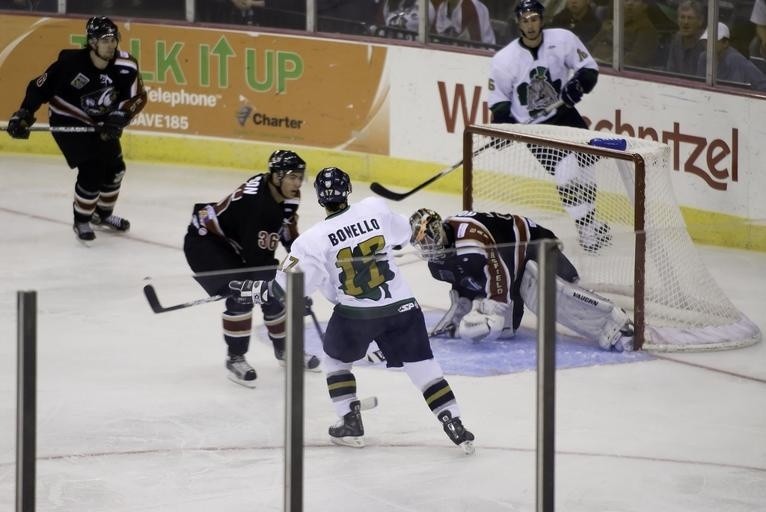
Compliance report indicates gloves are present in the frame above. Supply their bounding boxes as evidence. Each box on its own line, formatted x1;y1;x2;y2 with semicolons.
561;79;583;106
96;121;122;138
8;111;36;139
230;279;268;306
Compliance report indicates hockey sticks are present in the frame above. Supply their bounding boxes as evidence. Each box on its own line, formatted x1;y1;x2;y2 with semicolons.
365;324;458;364
369;100;562;201
144;277;229;314
311;310;377;411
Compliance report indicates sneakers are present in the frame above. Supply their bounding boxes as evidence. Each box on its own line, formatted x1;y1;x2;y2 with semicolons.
273;351;320;367
328;401;364;438
74;221;95;241
580;232;600;250
226;354;257;380
437;411;474;446
103;215;129;231
596;222;611;243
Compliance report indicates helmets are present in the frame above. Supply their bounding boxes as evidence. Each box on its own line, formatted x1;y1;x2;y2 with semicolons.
314;167;352;202
515;1;546;18
410;210;445;263
86;16;118;42
269;151;305;171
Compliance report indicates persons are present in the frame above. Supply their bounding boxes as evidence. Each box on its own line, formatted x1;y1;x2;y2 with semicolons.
224;168;475;454
410;208;634;354
547;0;600;45
370;1;434;43
432;0;495;52
199;0;274;28
582;0;656;66
747;1;766;61
9;16;147;241
666;0;708;75
487;0;611;251
182;151;321;387
697;23;766;92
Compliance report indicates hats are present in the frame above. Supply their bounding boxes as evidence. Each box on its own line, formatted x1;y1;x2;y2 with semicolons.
700;22;730;41
